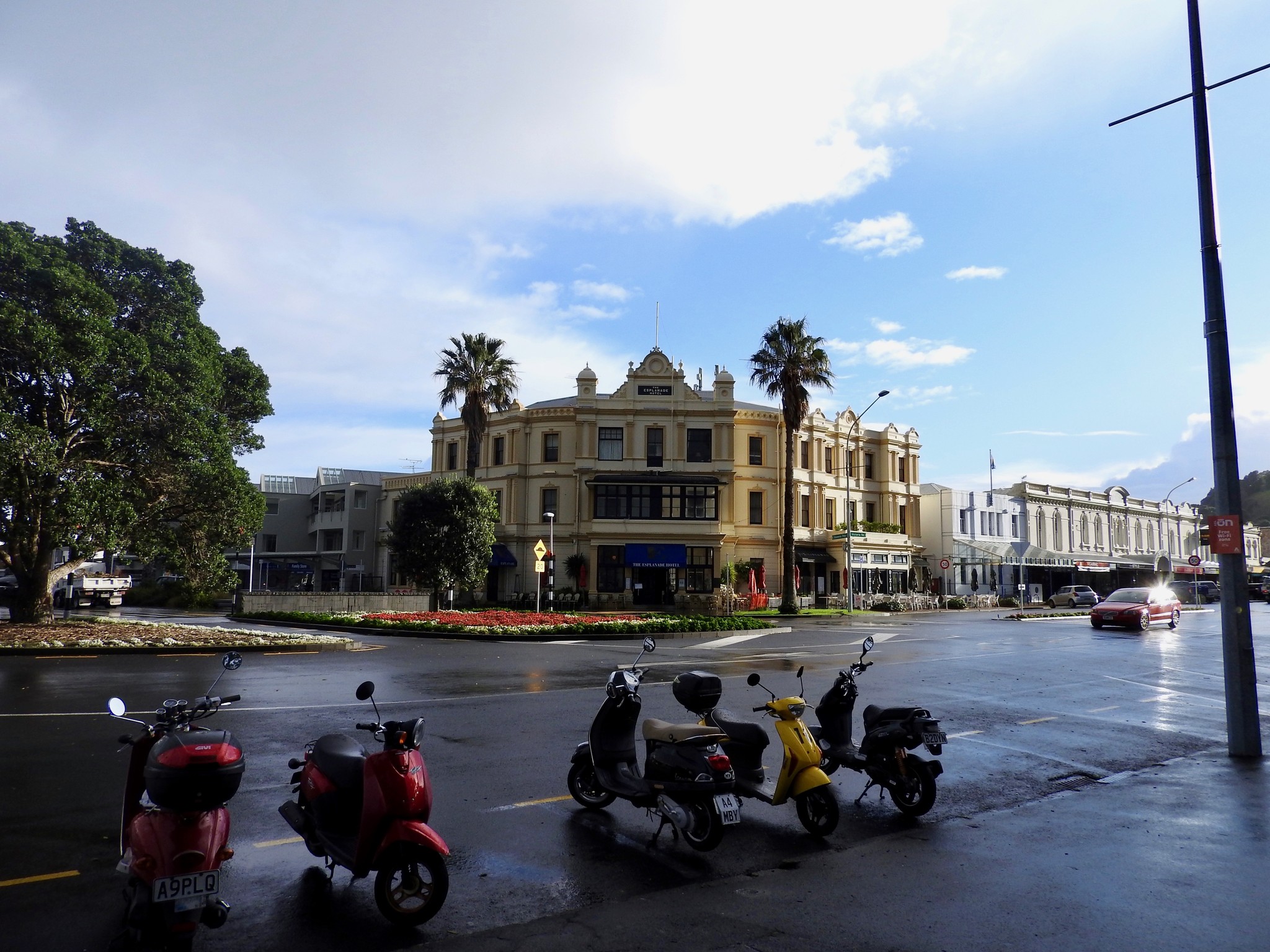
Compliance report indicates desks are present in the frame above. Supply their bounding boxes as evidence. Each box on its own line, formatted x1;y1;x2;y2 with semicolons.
705;595;717;609
798;597;812;609
678;595;688;607
947;595;957;600
819;597;838;609
734;597;746;611
768;597;811;608
921;596;932;610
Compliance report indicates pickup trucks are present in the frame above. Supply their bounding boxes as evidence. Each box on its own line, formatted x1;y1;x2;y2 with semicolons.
1162;581;1209;604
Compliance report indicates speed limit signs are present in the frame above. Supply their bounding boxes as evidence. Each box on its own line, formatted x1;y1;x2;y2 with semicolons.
1188;555;1201;566
940;559;949;569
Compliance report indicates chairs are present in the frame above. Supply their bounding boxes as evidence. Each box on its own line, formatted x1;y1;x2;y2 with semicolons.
506;593;634;611
673;594;681;608
720;584;751;613
963;592;1000;608
831;593;951;611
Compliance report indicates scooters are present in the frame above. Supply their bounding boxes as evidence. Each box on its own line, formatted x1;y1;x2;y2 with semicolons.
106;650;243;952
567;635;744;851
672;665;840;837
806;635;947;816
278;681;452;929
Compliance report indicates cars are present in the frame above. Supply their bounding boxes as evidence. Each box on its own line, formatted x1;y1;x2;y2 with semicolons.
1188;581;1221;603
1247;574;1270;604
1048;585;1098;609
1090;586;1182;631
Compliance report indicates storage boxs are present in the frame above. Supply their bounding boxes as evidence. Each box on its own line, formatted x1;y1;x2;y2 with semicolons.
673;670;722;714
143;729;246;811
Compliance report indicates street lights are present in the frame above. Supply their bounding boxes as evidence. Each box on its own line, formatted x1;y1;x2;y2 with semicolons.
543;512;554;611
1166;477;1196;580
846;390;890;612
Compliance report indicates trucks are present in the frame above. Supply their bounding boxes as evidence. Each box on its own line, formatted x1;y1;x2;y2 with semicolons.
50;561;132;610
